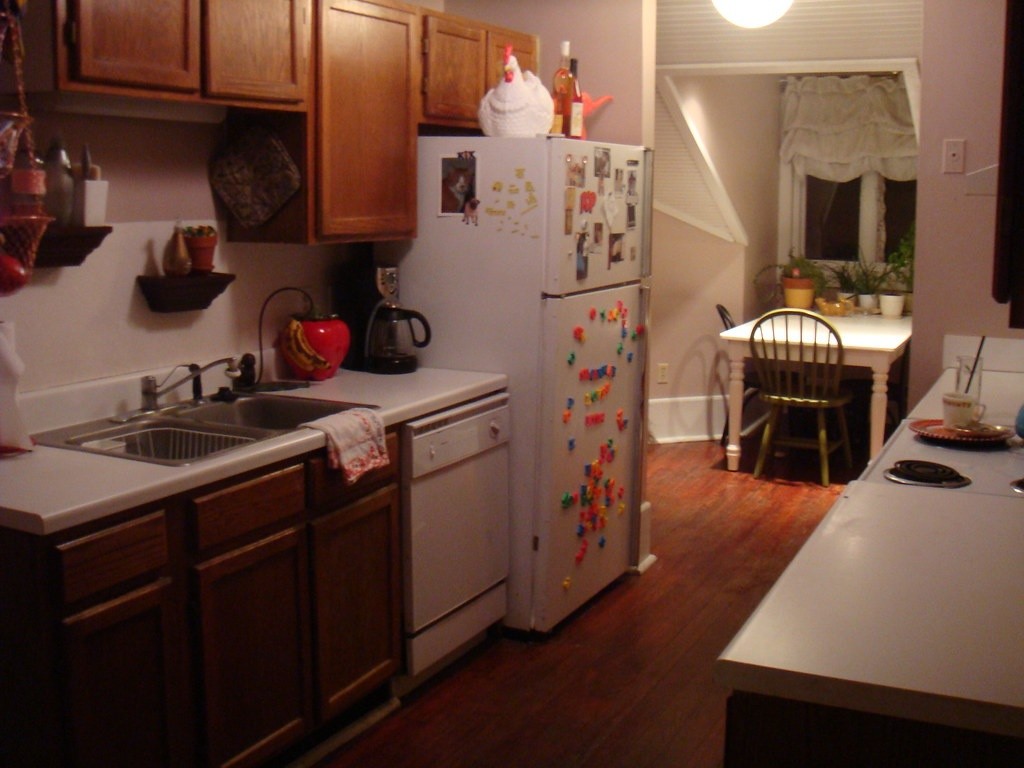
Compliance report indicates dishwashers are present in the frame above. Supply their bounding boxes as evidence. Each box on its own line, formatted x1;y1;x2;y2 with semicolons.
401;394;511;677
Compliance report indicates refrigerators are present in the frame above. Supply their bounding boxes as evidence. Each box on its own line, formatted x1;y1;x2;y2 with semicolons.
393;136;655;631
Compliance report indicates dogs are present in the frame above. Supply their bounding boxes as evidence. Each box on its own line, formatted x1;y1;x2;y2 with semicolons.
462;198;481;227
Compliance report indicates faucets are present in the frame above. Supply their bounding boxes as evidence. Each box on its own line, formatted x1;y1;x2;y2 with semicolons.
140;357;242;409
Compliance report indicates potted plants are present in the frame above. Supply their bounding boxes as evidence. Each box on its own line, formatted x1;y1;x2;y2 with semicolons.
836;268;857;306
855;270;879;309
780;257;824;305
882;277;905;312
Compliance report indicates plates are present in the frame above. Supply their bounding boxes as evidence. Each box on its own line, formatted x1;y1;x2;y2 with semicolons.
908;419;1015;442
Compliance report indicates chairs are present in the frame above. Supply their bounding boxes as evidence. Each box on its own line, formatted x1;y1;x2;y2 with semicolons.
741;307;853;487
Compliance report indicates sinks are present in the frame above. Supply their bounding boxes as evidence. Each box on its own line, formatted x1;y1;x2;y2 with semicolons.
160;393;381;436
28;417;277;468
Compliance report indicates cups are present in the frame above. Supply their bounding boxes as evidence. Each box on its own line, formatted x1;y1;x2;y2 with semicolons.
956;353;984;417
944;392;985;432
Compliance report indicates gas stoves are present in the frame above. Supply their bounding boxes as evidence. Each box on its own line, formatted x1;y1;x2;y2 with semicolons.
857;417;1024;499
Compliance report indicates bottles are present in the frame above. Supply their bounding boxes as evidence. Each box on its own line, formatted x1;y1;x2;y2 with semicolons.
562;59;582;139
10;145;46;216
551;41;572;134
43;139;75;229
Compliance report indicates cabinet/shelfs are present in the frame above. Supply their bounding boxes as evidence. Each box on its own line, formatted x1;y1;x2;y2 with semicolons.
0;0;538;244
0;434;407;767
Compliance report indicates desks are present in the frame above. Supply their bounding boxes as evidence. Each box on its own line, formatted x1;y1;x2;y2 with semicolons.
723;314;911;470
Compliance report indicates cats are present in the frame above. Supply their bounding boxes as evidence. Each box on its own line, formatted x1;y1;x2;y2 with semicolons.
442;165;471;213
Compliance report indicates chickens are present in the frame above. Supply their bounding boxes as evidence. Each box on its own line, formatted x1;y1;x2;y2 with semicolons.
476;43;556;139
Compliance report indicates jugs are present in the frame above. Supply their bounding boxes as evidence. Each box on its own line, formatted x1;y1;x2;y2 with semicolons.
815;298;855;316
366;304;432;374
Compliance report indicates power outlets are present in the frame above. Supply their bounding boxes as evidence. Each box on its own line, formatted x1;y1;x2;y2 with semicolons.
659;364;668;383
